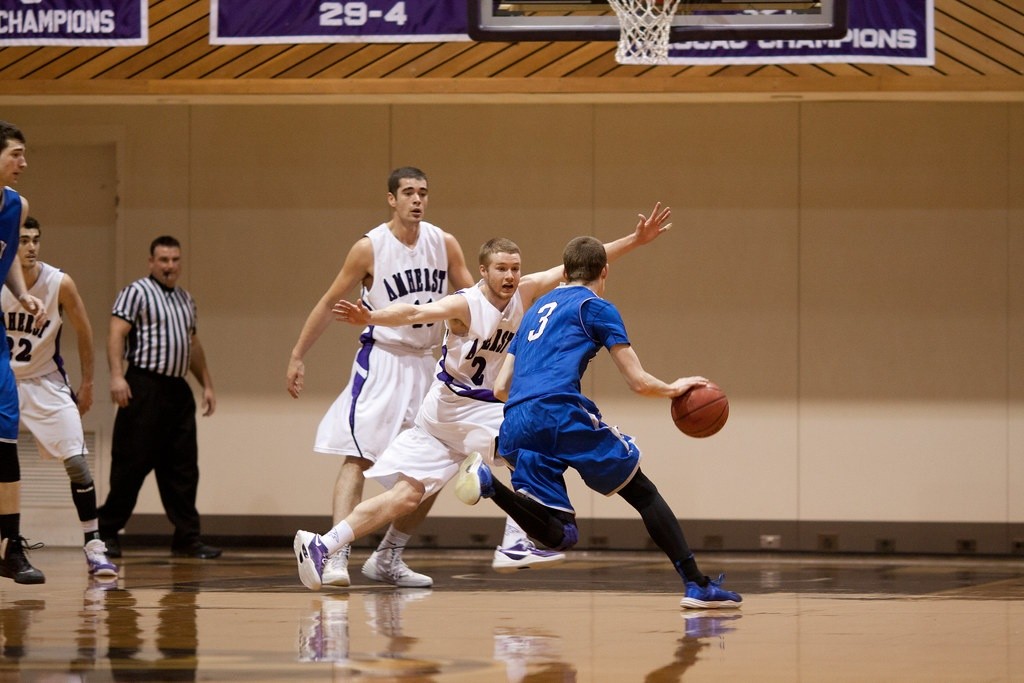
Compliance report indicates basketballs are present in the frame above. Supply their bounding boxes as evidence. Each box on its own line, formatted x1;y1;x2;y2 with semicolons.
671;380;730;439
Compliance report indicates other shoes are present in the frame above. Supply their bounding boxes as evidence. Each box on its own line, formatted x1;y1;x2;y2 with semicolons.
93;537;123;556
173;535;222;561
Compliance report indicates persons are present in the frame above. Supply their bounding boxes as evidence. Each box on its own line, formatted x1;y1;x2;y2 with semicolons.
0;122;118;585
97;236;222;558
286;166;743;608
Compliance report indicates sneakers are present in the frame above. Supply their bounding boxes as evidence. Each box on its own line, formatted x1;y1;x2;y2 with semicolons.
318;543;354;586
0;535;47;585
293;528;332;592
455;448;496;504
361;547;435;587
492;538;566;573
82;537;121;578
678;579;746;608
